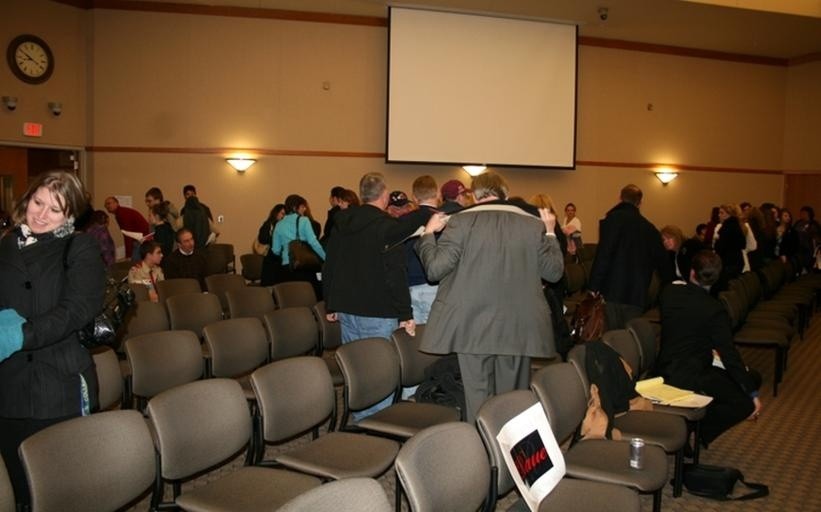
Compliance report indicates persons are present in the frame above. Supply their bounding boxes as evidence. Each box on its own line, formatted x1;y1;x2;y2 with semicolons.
1;169;213;511
257;171;582;425
597;178;820;459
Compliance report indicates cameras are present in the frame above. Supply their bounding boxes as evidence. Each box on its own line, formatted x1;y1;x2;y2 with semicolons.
390;191;407;207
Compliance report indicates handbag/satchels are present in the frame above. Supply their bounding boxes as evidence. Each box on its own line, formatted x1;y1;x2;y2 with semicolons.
415;374;465;407
252;222;274;257
63;231;136;345
288;214;323;273
423;353;460;382
570;294;605;342
671;464;769;502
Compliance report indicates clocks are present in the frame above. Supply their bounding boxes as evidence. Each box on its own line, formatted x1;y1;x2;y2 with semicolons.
7;32;56;85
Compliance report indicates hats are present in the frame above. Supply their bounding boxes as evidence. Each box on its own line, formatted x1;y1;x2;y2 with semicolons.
388;190;414;206
441;179;472;202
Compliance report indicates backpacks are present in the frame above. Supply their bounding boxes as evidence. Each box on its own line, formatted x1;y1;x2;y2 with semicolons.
584;342;634;415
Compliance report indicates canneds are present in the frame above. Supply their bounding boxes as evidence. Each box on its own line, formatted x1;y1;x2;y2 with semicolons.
630;438;645;469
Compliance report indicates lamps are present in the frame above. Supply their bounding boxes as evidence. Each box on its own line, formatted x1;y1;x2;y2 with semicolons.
655;170;677;184
461;163;487;178
227;157;257;172
